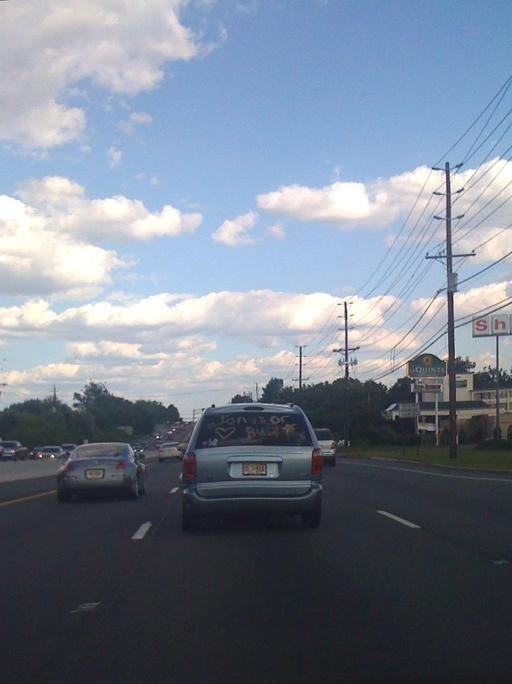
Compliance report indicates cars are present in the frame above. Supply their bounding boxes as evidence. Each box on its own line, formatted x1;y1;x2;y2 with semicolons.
0;420;187;461
56;439;148;501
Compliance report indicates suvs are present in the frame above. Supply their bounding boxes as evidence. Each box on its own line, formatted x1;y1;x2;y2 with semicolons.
177;401;327;530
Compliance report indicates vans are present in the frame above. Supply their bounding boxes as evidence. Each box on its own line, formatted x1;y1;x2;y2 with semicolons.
309;426;339;468
